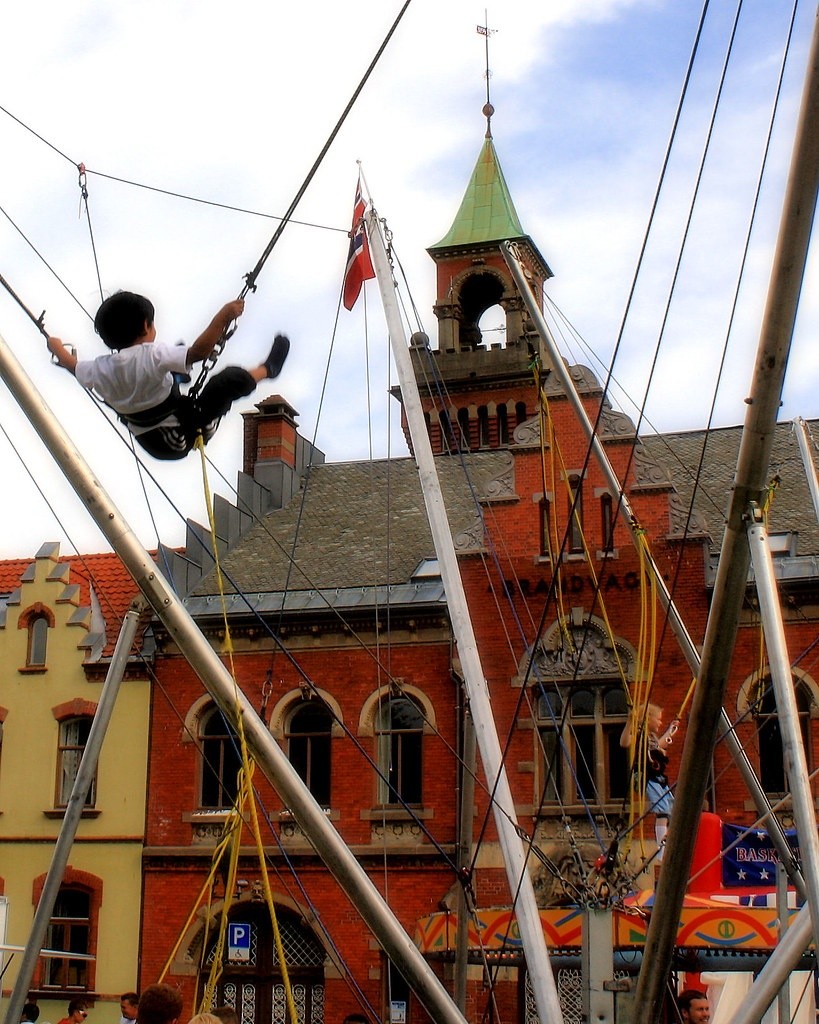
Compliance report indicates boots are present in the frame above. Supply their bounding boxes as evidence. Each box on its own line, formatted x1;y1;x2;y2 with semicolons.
655;826;667;861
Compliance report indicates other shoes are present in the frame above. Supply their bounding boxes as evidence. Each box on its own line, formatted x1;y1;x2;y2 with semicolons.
263;336;290;377
173;344;191;383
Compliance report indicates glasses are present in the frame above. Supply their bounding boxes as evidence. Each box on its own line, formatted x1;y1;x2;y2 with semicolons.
78;1009;87;1017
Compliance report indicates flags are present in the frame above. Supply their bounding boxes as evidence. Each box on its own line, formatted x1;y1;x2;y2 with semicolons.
343;176;376;311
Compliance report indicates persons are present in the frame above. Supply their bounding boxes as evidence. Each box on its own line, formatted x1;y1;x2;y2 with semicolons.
16;983;239;1024
46;291;291;461
619;702;684;861
678;988;711;1024
